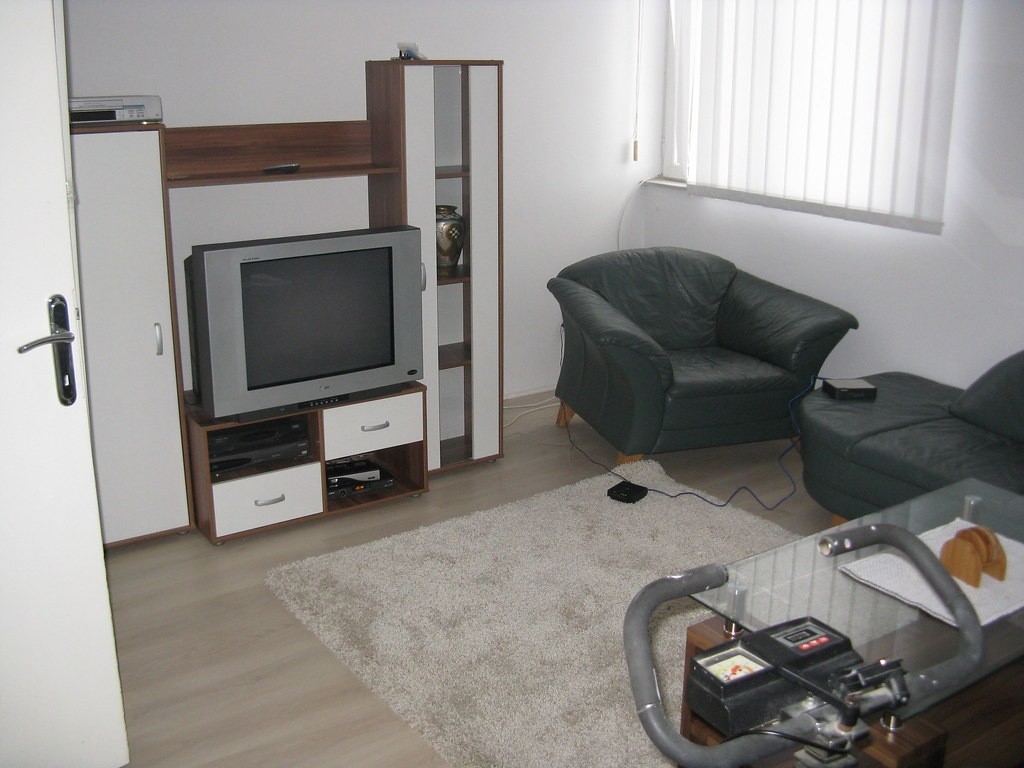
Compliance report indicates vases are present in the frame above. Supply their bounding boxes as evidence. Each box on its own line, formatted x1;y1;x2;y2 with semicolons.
436;205;464;277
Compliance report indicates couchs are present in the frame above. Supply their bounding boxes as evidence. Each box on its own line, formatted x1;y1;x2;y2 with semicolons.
546;245;858;466
798;350;1024;528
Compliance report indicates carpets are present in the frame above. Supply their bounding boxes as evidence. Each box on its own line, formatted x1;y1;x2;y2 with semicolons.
262;458;919;768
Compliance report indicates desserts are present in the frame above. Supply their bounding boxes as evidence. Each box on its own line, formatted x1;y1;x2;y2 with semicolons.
940;525;1007;587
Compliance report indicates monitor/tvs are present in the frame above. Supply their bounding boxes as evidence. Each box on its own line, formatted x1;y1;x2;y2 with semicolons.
183;225;421;424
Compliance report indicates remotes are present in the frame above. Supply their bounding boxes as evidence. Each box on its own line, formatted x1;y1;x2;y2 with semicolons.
263;164;300;174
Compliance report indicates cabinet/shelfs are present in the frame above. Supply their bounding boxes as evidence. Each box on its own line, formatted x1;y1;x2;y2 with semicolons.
70;58;505;550
183;381;430;547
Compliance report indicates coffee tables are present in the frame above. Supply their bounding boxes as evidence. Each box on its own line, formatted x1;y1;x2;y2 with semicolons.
679;479;1024;768
